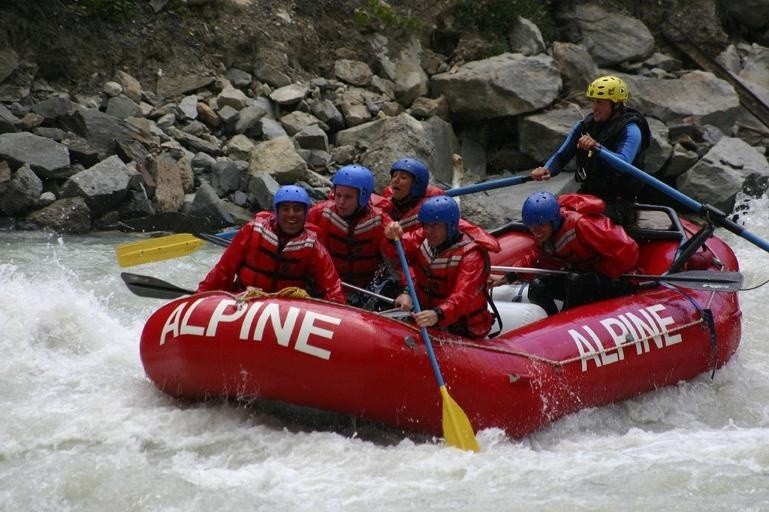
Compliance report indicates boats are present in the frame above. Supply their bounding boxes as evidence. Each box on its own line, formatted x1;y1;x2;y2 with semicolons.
140;205;743;438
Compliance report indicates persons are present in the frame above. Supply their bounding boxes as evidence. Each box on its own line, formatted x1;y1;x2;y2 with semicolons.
305;165;418;311
384;194;504;341
523;191;640;318
532;76;652;230
192;185;347;304
371;158;443;235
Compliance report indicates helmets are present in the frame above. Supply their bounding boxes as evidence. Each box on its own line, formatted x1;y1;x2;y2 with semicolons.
520;190;562;235
333;163;374;218
417;195;460;251
585;73;629;112
390;157;429;208
273;185;310;236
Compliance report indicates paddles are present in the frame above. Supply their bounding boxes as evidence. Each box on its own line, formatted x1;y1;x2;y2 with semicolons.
393;235;481;453
591;143;769;253
490;266;745;293
191;230;401;307
115;173;533;268
120;271;195;299
